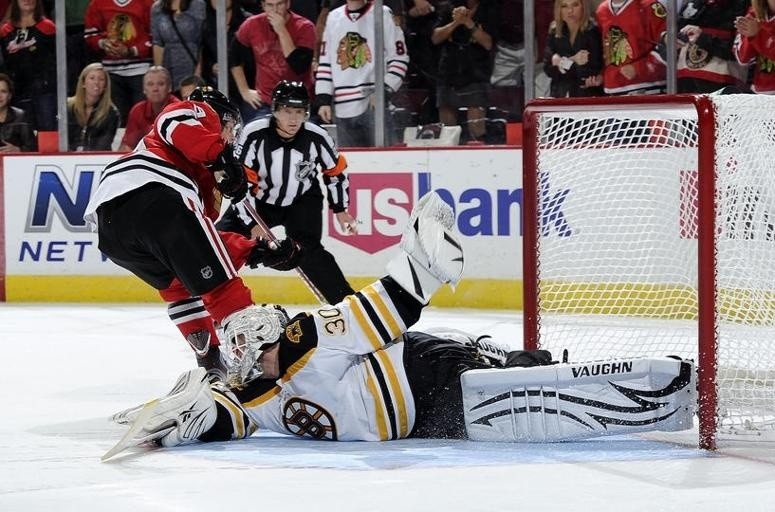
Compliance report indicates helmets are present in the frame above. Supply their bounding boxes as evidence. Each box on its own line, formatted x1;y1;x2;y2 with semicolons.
271;78;311;123
187;84;245;147
218;303;290;390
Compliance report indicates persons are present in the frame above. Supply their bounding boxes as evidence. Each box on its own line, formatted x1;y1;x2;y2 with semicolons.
214;79;357;306
151;217;552;447
82;84;302;385
1;2;775;154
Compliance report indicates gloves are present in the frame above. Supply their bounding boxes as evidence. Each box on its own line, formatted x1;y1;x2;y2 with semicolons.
203;143;248;204
245;235;304;271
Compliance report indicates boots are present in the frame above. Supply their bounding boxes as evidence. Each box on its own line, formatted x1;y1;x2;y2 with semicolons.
186;328;231;383
